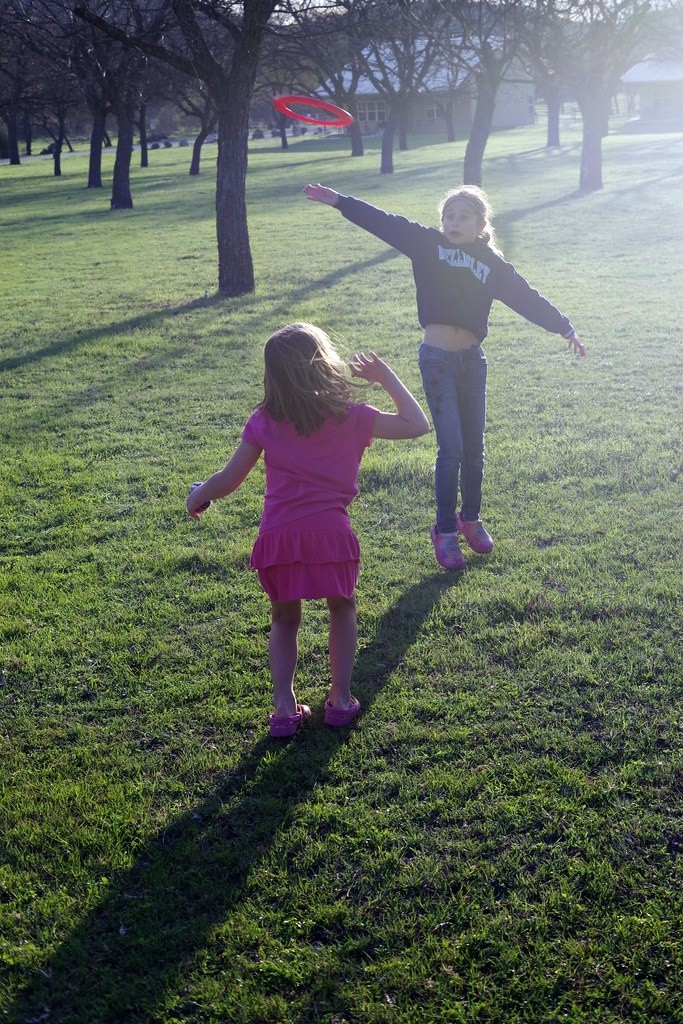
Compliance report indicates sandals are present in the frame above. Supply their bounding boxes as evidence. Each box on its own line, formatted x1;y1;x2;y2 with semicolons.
430;524;464;568
324;694;361;727
268;704;311;737
456;511;493;553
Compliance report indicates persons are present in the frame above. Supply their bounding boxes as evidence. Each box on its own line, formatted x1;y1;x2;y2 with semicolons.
302;182;585;567
186;323;428;735
